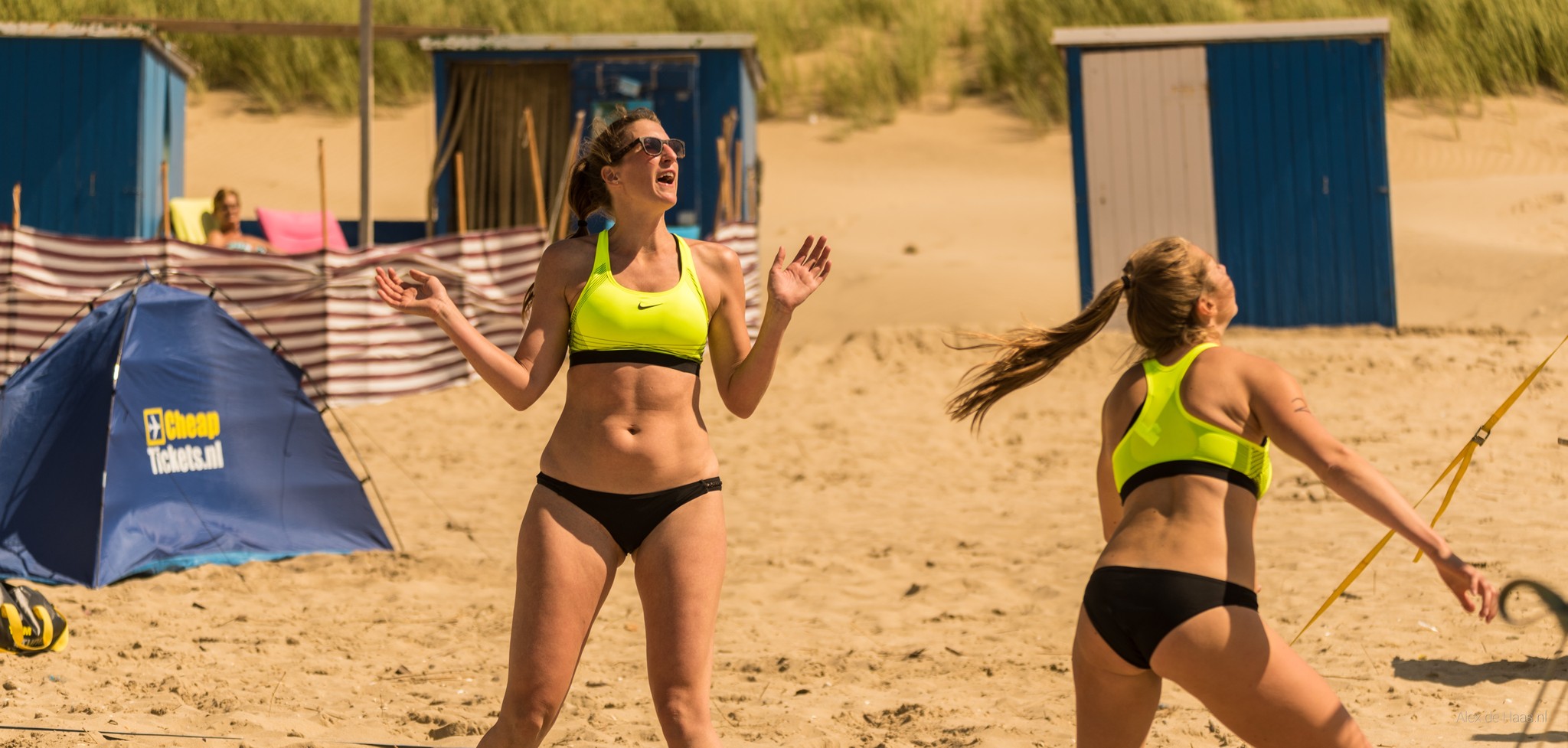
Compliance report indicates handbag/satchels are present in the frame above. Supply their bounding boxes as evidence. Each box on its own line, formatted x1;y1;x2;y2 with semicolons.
0;580;69;655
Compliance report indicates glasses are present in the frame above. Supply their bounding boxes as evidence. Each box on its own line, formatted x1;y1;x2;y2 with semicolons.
611;138;685;163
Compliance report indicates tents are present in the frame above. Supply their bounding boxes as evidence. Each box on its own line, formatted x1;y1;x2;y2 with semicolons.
0;267;406;588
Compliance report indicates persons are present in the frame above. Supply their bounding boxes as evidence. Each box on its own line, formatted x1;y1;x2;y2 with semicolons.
942;235;1500;748
375;106;833;748
204;190;276;257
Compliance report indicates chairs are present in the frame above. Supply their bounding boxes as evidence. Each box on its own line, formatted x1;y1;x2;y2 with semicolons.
256;209;349;256
169;196;214;244
